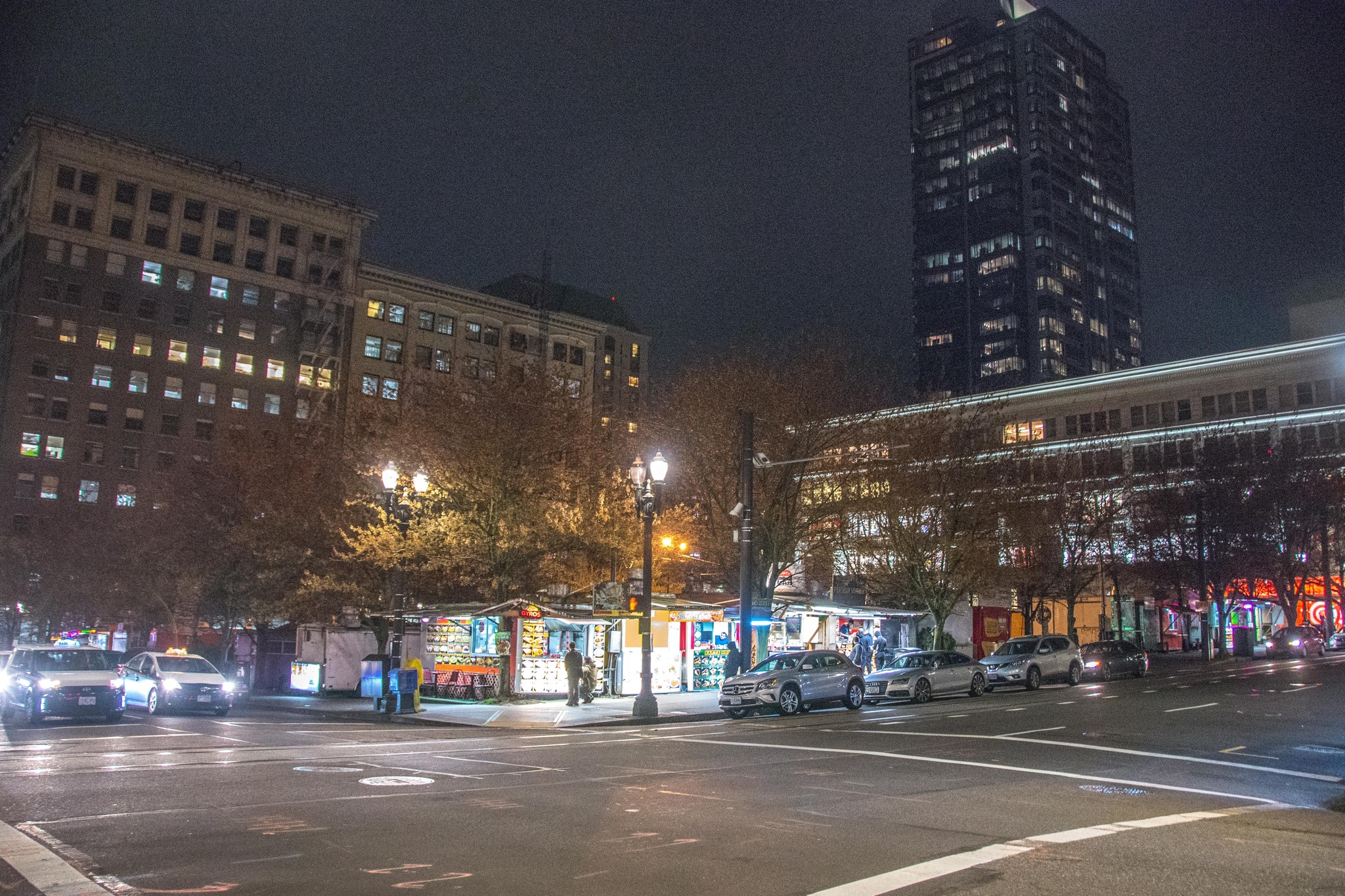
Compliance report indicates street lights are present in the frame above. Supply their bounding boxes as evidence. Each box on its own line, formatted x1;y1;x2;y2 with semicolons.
380;458;430;715
629;448;668;715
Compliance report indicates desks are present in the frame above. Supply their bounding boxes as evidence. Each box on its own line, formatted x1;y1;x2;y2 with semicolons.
460;674;483;701
427;671;446;698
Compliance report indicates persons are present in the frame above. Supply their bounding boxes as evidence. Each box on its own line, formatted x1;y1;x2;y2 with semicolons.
715;632;730;646
861;627;873;675
854;628;869;674
839;619;855;640
578;656;599;703
723;641;739;681
871;631;887;671
848;636;864;671
564;641;582;706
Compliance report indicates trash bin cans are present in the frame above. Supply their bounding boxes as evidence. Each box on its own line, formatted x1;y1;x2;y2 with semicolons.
404;658;427;712
360;654;391;710
894;647;922;668
387;667;418;714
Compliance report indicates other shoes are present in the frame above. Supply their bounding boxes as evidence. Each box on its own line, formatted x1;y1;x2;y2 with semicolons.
588;695;594;703
582;696;590;703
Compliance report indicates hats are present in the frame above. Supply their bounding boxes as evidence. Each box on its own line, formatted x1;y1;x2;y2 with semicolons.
847;619;854;625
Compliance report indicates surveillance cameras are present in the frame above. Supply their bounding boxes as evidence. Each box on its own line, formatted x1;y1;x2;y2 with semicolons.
729;503;743;517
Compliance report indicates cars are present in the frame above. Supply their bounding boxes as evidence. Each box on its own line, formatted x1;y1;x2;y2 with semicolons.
1080;639;1149;682
118;647;233;716
1265;626;1326;660
0;650;13;676
1329;626;1345;652
864;650;990;706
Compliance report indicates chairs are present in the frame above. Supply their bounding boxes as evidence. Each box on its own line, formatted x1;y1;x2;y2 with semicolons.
911;658;921;666
436;669;471;699
828;659;838;665
784;659;795;667
473;669;495;699
420;668;434;696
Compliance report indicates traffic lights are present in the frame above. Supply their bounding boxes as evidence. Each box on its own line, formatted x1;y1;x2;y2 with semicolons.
626;594;641;613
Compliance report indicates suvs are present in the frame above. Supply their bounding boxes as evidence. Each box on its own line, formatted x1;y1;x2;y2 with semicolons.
718;650;866;719
978;633;1085;693
0;645;124;725
